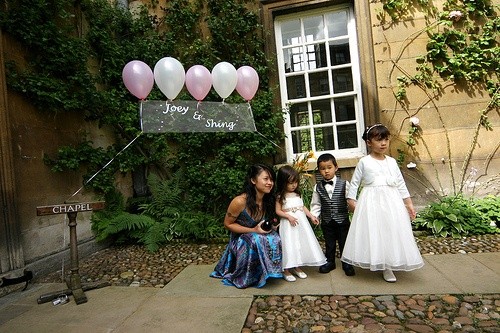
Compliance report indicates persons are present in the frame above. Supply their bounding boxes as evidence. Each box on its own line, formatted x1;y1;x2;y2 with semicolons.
275;165;319;282
309;153;355;276
340;124;425;282
208;162;283;289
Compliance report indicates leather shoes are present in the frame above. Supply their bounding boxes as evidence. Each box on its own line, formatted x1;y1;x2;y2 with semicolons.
319;261;336;273
342;262;355;276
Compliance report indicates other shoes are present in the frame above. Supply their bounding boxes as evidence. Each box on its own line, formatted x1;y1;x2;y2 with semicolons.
383;270;396;282
292;269;307;278
283;273;296;281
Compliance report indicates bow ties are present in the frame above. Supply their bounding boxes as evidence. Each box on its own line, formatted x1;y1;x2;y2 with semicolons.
322;180;333;186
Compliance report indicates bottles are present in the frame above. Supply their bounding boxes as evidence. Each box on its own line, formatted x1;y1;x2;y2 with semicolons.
261;218;277;231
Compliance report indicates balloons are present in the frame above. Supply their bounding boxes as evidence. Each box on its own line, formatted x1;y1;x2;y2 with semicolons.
212;62;237;103
154;57;185;102
236;66;259;102
185;65;213;102
122;60;153;101
